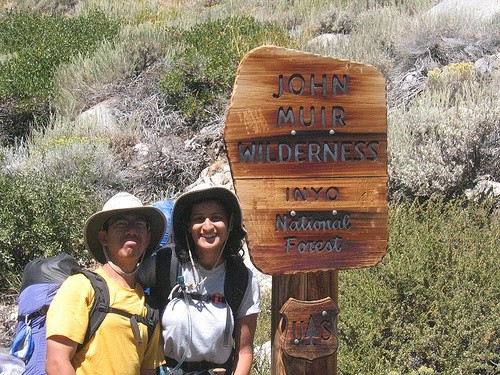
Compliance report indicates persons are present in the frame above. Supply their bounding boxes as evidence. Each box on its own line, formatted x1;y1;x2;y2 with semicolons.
46;193;167;375
134;184;260;375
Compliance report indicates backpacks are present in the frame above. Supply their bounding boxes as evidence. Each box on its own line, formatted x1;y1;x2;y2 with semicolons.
137;246;249;375
10;251;160;375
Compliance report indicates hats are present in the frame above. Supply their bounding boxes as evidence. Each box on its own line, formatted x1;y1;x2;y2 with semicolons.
171;183;247;244
83;191;166;264
151;199;175;246
